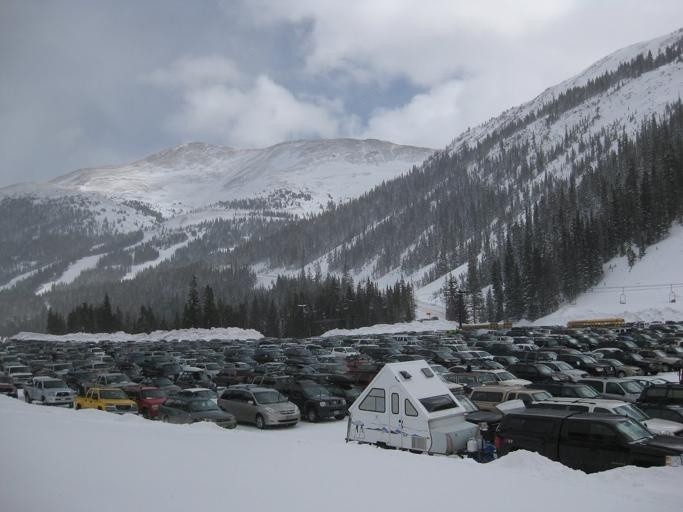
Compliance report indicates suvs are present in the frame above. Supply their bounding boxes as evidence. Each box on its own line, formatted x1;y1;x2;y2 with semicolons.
464;405;682;474
